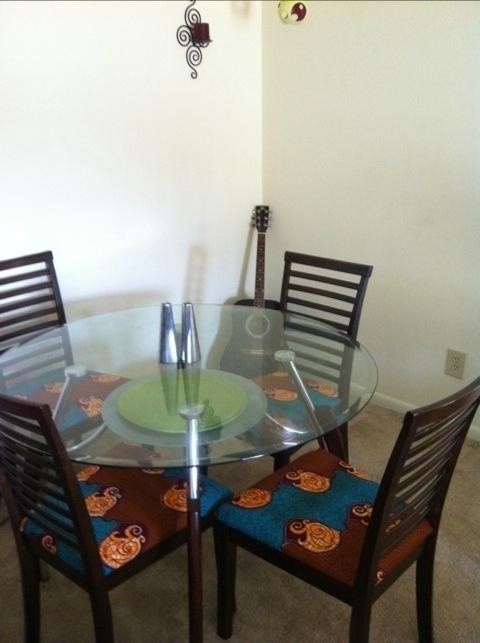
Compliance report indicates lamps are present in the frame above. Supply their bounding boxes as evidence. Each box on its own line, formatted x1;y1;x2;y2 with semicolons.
176;0;211;76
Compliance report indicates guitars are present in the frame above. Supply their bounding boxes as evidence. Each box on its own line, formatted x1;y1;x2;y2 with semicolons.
220;206;281;380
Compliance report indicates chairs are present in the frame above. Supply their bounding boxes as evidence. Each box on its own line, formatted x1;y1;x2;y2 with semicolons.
1;249;168;585
209;371;480;642
194;250;373;479
1;390;241;642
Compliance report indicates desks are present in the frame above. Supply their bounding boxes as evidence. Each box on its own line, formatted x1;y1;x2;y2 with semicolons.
2;299;384;642
